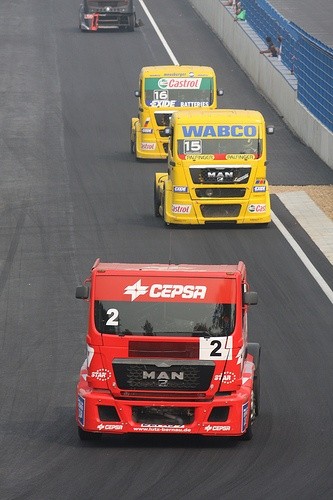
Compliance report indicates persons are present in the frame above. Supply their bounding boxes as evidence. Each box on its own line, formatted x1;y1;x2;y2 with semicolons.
259;36;283;57
222;0;247;21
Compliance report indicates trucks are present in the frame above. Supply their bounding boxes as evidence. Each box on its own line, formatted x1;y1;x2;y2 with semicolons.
79;0;136;32
131;65;225;160
75;257;261;441
152;109;275;227
